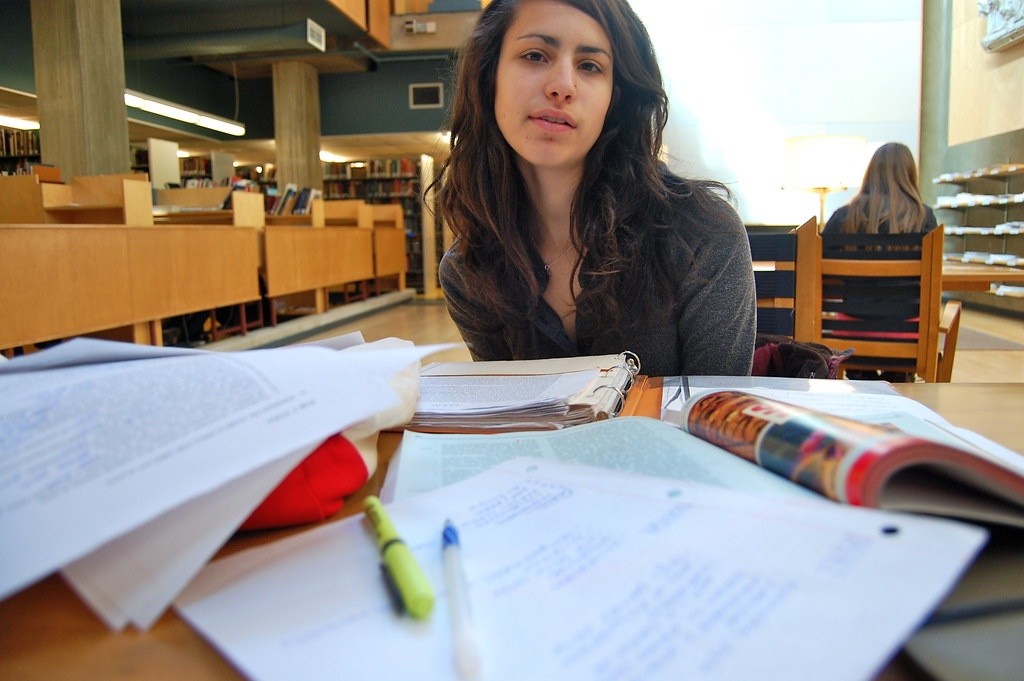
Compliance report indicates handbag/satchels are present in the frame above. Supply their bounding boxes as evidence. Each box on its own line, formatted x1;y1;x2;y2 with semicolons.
753;335;853;379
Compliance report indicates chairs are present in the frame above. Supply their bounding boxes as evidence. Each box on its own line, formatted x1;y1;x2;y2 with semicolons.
746;216;824;344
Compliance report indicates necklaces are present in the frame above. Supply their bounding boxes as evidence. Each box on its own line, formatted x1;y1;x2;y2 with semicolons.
532;239;579;279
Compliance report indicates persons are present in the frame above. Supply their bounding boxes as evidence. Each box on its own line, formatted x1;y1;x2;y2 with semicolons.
423;1;758;375
821;142;934;383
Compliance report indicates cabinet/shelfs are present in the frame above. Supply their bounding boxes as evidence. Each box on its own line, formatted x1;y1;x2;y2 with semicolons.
932;162;1024;319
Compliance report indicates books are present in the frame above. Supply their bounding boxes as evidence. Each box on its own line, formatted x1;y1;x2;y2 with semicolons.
1;127;422;290
389;353;1024;527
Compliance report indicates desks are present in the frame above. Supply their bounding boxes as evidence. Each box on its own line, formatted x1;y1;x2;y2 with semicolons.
1;376;1024;681
0;174;403;359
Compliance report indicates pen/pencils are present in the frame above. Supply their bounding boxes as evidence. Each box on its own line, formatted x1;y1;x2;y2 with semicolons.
441;519;481;681
365;493;434;619
